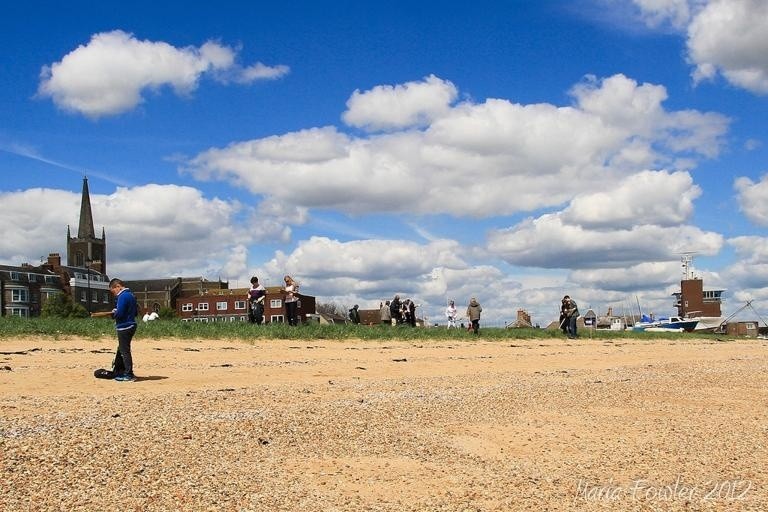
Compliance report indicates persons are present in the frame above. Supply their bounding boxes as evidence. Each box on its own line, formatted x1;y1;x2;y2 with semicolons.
108;278;139;381
351;305;360;325
381;295;417;327
148;310;161;320
564;294;580;339
445;300;458;329
465;298;482;332
247;277;267;324
281;276;300;326
142;312;150;323
559;298;570;334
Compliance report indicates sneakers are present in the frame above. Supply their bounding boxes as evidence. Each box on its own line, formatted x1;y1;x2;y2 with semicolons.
115;373;132;381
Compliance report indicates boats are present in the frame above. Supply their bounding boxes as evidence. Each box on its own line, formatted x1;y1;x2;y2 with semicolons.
624;315;700;333
